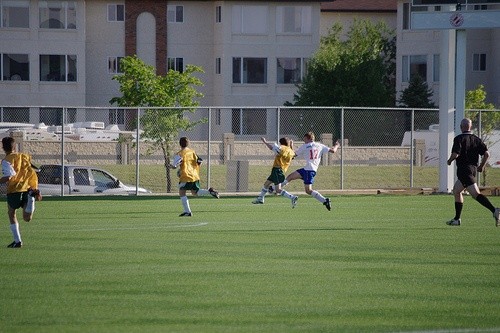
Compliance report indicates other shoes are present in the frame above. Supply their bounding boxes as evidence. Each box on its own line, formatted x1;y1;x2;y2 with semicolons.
8;241;22;248
208;187;220;199
252;198;264;204
493;208;500;227
291;196;298;208
446;218;461;226
179;212;192;217
323;197;331;211
26;187;40;197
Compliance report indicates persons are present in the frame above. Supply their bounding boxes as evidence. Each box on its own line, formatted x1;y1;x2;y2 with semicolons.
252;137;298;208
268;132;340;211
173;137;220;217
446;118;500;227
0;137;42;248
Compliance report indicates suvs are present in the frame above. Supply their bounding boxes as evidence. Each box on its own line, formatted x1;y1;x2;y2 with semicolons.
37;163;152;196
